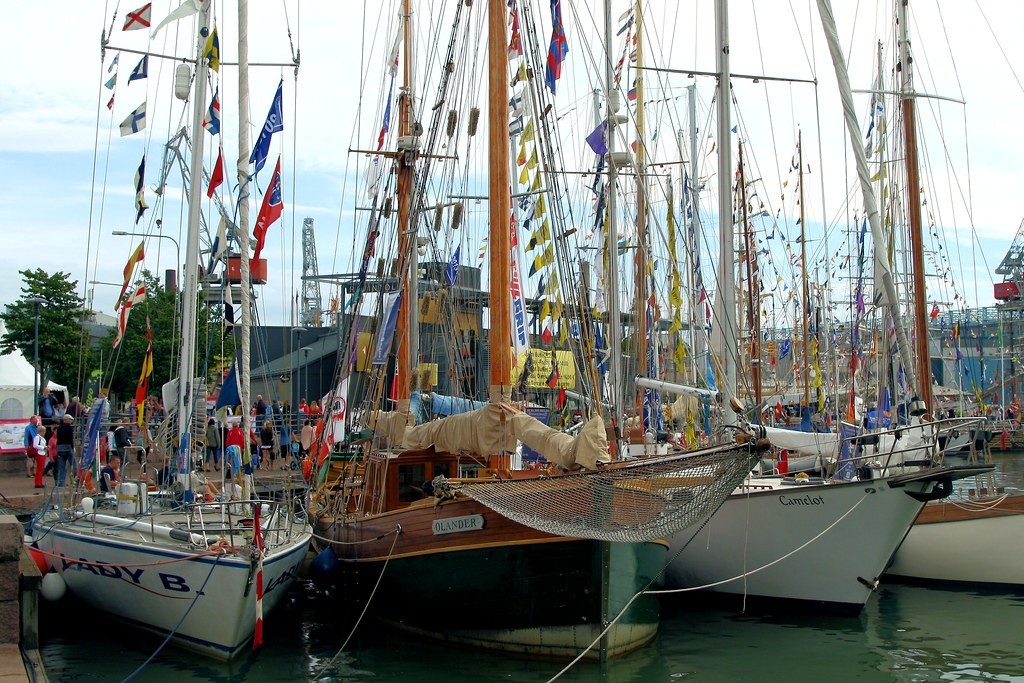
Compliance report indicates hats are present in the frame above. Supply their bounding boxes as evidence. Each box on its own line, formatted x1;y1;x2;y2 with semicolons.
63;414;74;420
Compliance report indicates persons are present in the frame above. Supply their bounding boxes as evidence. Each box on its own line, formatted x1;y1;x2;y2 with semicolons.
23;388;324;496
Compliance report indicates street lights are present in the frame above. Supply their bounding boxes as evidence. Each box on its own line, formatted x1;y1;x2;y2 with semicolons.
111;231;181;382
25;296;51;417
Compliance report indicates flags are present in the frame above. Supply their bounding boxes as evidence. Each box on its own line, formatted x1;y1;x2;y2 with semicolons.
101;0;1024;518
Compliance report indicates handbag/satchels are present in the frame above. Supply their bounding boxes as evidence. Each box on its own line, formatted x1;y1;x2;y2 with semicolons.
27;436;41;458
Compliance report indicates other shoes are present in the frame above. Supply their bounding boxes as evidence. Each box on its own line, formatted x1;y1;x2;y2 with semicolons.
26;474;34;478
35;484;45;488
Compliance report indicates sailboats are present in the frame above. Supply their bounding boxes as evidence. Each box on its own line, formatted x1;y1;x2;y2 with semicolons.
22;0;1024;668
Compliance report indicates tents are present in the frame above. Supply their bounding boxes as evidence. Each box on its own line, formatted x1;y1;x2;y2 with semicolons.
0;321;70;421
